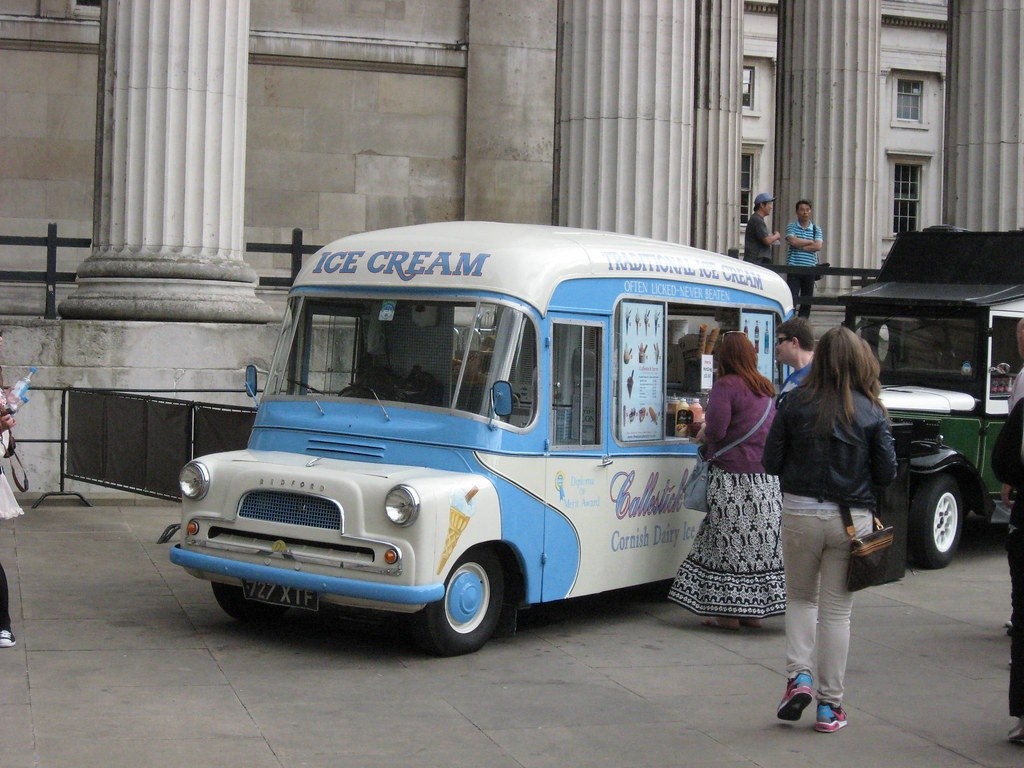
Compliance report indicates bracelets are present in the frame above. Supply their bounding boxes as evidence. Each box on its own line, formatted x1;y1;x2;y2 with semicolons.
801;245;804;250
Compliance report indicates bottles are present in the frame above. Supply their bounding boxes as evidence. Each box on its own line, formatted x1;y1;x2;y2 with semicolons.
764;321;769;354
8;367;37;402
754;320;759;353
674;398;689;437
667;394;680;435
744;320;748;336
6;393;30;415
687;398;703;436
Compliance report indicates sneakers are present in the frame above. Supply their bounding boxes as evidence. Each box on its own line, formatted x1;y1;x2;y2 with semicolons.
0;630;16;648
815;703;848;732
777;672;813;721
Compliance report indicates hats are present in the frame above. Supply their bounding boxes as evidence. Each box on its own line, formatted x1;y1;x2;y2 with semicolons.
755;193;776;205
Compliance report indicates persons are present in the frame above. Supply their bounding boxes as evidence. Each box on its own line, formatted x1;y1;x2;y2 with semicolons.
0;328;25;646
742;193;781;265
764;328;894;733
991;318;1024;743
451;334;513;425
775;316;815;409
784;201;823;320
667;331;786;630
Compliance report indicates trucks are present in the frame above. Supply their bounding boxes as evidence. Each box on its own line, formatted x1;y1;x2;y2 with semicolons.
844;223;1024;569
169;220;798;657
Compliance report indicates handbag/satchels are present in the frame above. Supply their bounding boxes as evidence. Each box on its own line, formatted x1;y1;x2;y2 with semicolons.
847;526;902;591
815;262;830;281
683;444;710;512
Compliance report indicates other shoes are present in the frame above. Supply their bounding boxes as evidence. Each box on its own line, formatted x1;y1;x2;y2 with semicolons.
1008;720;1023;739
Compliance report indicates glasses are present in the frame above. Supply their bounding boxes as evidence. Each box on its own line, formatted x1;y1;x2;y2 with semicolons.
722;330;747;342
777;337;791;344
